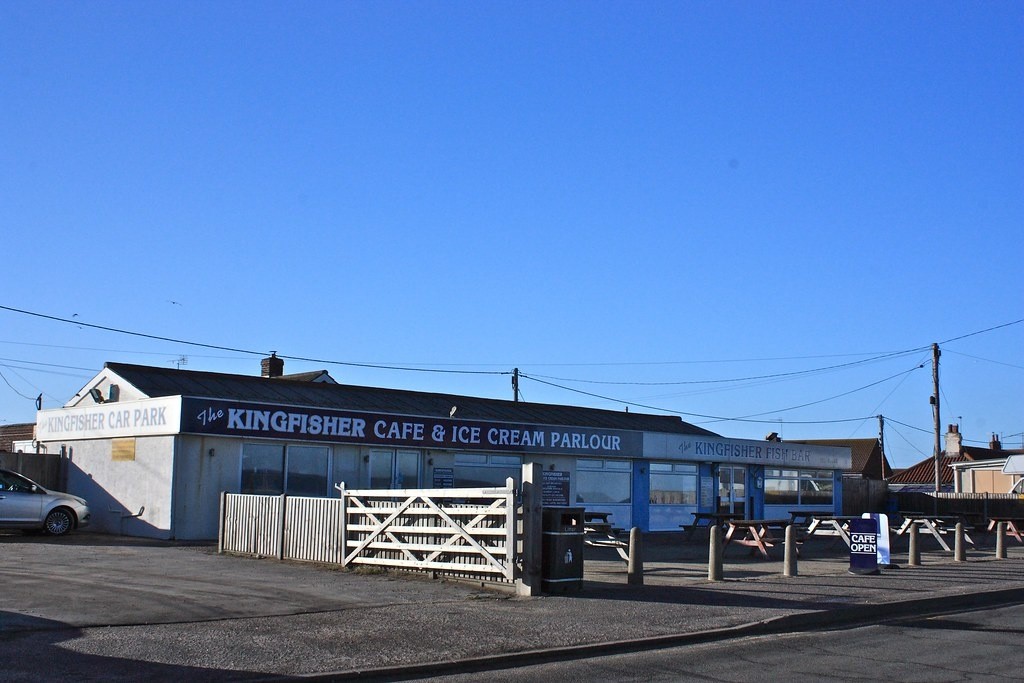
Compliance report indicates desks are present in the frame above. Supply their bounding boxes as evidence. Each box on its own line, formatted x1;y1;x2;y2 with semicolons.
786;510;834;531
891;515;979;552
806;515;853;548
689;513;745;533
720;518;796;559
984;517;1024;547
585;511;614;522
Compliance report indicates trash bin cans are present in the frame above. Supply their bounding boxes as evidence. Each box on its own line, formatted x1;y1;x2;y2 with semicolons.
541;505;585;593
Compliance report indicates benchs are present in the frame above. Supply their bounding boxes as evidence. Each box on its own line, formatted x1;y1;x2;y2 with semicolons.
584;523;616;536
681;524;710;535
732;536;804;553
928;526;973;535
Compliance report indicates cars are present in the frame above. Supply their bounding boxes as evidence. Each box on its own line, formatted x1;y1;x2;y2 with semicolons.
0;468;90;537
